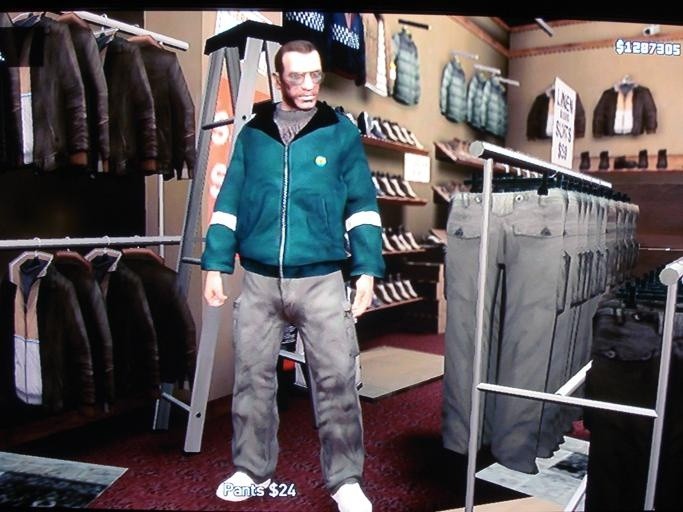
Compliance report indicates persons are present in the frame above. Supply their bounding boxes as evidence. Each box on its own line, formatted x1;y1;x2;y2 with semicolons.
201;41;385;512
